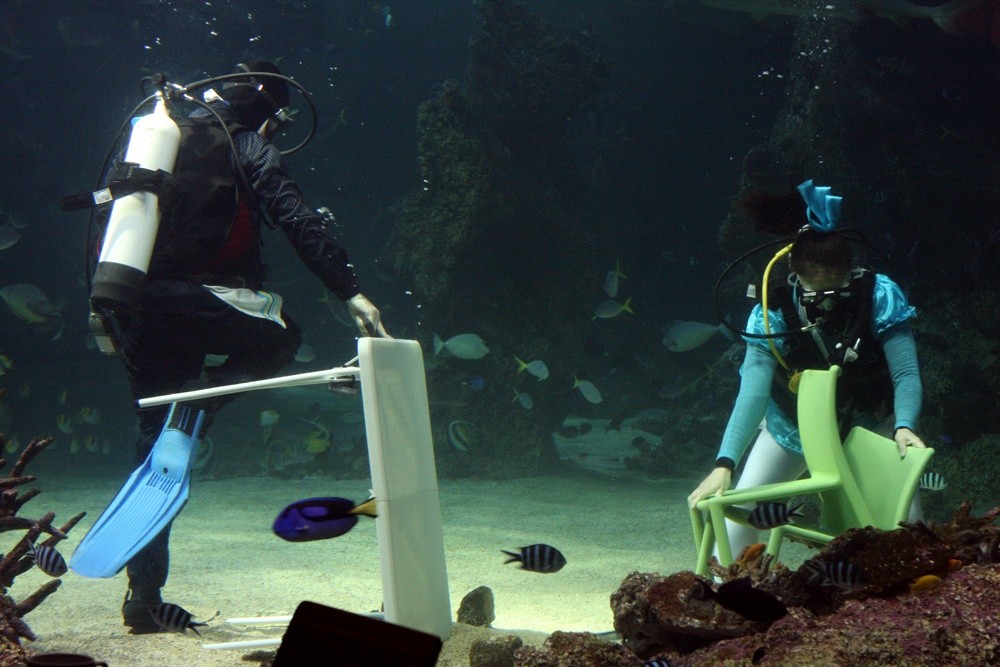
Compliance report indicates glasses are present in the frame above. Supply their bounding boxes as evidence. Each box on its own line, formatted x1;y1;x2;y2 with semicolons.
798;275;854;306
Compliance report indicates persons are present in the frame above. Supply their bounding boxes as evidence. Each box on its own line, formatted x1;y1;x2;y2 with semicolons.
686;182;928;590
60;52;400;632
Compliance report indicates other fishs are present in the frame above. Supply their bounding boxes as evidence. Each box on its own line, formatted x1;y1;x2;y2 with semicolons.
148;603;211;639
502;543;567;573
272;496;377;543
640;576;789;667
0;229;744;486
747;502;805;530
905;473;963;595
22;538;68;577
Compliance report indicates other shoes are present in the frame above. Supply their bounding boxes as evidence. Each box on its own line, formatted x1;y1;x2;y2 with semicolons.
121;599;207;636
72;379;217;581
